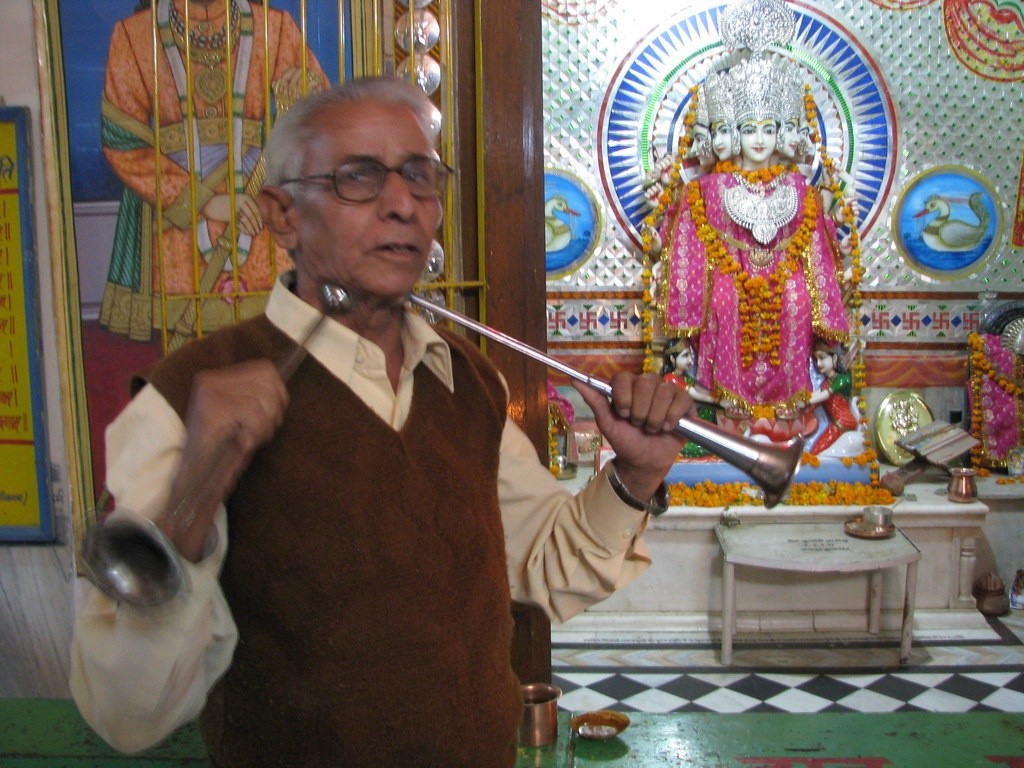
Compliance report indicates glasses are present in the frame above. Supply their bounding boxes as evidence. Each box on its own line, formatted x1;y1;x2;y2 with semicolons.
277;155;454;203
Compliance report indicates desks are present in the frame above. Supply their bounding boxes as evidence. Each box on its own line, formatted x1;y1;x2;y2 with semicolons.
712;522;919;668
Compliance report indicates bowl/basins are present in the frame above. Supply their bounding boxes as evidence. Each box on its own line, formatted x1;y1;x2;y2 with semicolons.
571;710;630;741
863;506;893;527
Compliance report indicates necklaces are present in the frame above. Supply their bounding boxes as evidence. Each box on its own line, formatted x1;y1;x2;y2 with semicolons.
725;171;797;245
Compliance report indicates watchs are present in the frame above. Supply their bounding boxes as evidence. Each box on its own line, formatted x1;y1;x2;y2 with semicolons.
607;461;670;516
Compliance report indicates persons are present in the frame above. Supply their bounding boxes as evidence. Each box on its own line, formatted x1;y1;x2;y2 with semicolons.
66;76;695;768
641;114;861;410
810;343;857;452
658;336;731;458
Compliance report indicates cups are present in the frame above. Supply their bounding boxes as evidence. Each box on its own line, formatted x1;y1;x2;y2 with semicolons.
517;682;562;747
